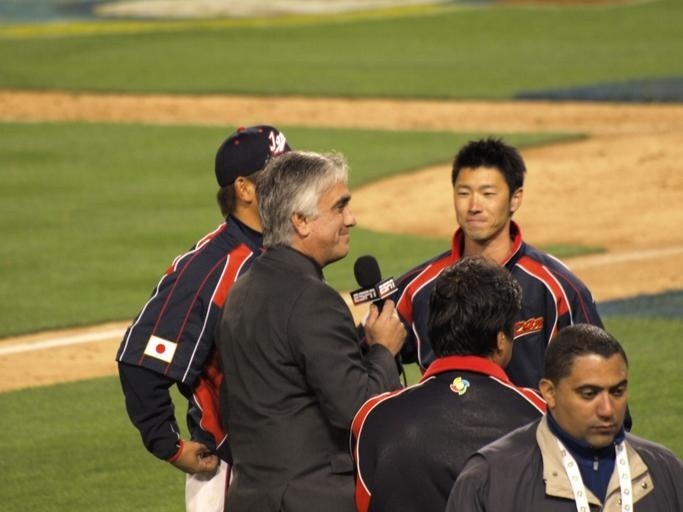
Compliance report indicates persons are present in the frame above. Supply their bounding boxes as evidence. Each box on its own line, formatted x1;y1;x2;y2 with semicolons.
391;136;632;432
445;323;683;512
349;256;548;512
217;148;407;511
115;125;294;512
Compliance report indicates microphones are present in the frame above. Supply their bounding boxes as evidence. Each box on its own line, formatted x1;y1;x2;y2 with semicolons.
350;255;404;366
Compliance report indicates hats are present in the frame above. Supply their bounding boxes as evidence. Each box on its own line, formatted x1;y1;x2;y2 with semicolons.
213;125;291;185
